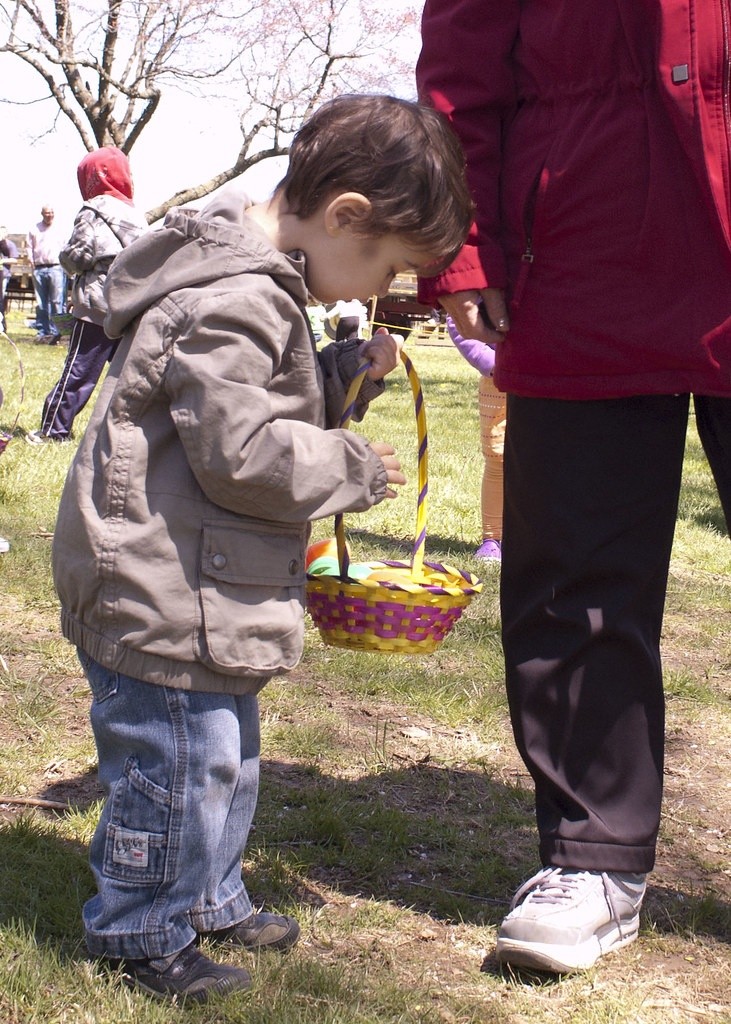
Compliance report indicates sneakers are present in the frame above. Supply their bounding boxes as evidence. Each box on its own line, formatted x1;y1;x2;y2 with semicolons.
495;866;647;974
90;944;251;1008
211;914;301;954
25;430;72;445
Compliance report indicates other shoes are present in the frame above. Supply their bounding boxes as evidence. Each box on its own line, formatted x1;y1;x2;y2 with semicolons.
474;538;501;562
0;538;10;552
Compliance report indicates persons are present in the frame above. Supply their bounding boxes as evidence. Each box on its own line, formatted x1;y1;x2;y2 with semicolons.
51;96;471;1009
445;310;506;559
26;147;150;448
26;206;70;345
416;0;731;976
0;225;17;316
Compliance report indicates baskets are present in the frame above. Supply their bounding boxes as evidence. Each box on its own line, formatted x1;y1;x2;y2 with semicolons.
0;332;24;456
52;303;78;335
306;347;482;656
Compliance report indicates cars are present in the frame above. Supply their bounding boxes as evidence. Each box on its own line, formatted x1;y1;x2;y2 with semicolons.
5;234;35;293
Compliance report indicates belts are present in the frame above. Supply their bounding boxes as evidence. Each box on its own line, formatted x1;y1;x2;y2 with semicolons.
36;264;59;268
